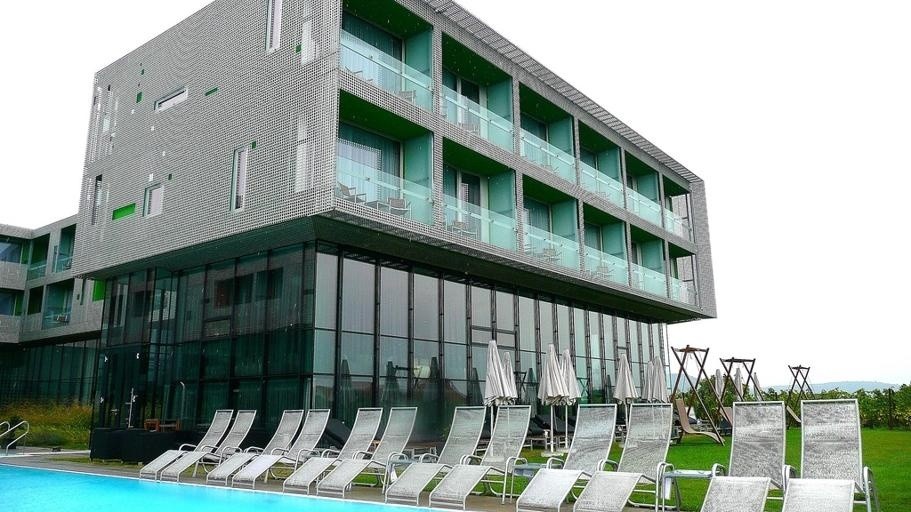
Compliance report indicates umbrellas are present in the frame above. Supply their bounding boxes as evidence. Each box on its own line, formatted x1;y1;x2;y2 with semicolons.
556;349;581;449
538;343;570;453
380;360;403;403
483;340;510;455
640;361;657;437
752;371;761;397
334;359;355;406
423;357;440;391
612;353;639;433
465;366;483;413
734;366;745;403
652;357;670;436
499;350;518;444
604;373;613;403
714;369;725;410
523;367;539;419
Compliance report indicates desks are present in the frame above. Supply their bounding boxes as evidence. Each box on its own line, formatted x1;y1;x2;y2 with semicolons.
381;453;438;493
662;462;728;512
303;450;342;487
501;457;567;503
221;447;260;480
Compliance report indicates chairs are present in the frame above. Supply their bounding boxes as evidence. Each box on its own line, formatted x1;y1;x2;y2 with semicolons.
384;405;487;505
285;407;384;495
701;401;797;512
340;62;567;178
429;405;533;510
206;410;304;485
233;409;332;489
515;403;620;512
781;396;876;512
315;406;419;498
473;413;734;456
339;180;616;279
572;402;682;512
138;411;235;480
160;410;257;482
